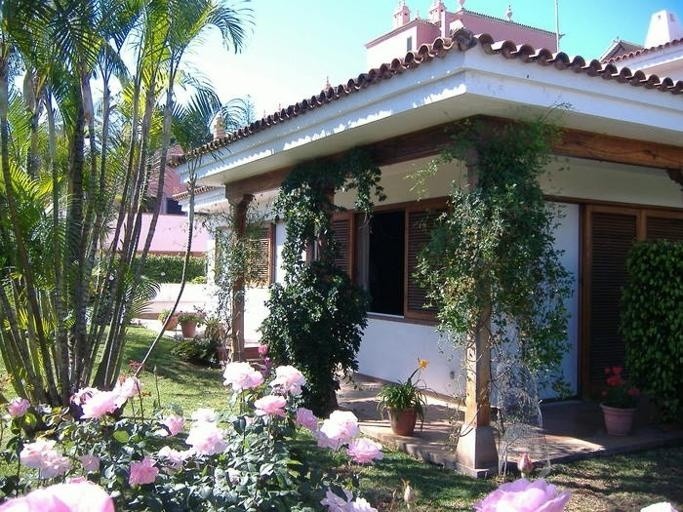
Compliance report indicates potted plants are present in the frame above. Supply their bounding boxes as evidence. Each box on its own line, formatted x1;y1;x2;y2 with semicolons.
158;309;178;330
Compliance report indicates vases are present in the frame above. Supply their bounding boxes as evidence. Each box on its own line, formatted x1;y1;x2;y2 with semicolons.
600;403;636;436
387;407;417;436
182;320;196;337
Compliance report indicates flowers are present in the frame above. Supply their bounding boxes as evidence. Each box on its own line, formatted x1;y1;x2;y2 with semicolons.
176;310;207;328
589;366;642;408
374;358;438;427
